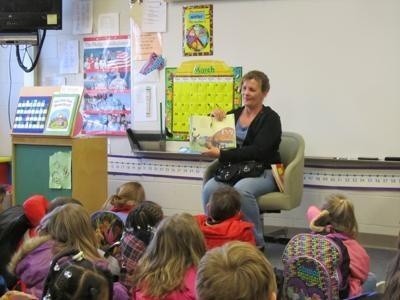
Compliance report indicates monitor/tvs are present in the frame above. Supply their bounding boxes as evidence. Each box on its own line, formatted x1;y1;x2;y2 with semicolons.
1;0;62;31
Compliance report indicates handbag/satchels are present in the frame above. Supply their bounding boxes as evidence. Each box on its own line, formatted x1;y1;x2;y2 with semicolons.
215;160;265;183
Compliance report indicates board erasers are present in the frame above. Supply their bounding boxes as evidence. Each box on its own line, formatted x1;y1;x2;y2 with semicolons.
384;156;399;161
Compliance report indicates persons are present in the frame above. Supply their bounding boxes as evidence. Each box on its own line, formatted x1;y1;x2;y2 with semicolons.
1;248;115;299
202;70;283;251
352;254;400;300
130;213;210;300
0;197;84;277
194;241;277;300
307;194;377;300
190;185;257;250
106;182;145;226
6;203;128;299
118;201;164;289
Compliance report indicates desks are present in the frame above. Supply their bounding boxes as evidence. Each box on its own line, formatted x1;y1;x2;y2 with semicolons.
9;133;109;216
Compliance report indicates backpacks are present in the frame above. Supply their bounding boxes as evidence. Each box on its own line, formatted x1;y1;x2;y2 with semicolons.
281;234;350;299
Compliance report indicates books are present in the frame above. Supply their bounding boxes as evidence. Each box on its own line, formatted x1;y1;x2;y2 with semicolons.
44;92;78;136
12;95;50;133
271;164;286;193
190;114;236;151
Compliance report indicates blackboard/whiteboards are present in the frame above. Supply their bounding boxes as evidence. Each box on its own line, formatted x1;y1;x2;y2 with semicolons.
127;0;399;169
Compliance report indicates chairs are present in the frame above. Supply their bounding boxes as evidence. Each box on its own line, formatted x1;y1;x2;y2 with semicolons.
202;131;305;247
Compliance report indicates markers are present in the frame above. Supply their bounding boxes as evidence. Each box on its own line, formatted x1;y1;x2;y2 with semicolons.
357;157;379;160
